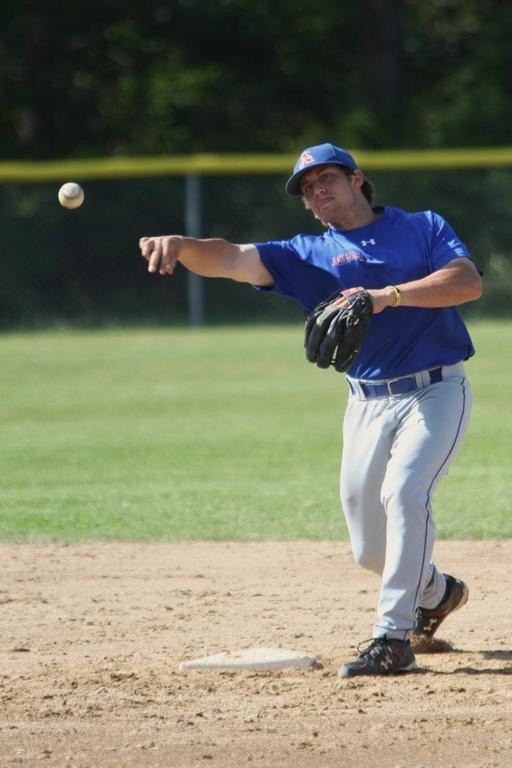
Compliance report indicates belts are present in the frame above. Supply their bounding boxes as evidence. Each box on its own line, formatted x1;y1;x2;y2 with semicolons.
346;367;442;398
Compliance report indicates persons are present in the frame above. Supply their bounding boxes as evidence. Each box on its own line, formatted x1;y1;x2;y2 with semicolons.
137;143;486;680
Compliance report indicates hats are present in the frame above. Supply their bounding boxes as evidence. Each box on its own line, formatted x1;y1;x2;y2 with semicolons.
285;144;359;196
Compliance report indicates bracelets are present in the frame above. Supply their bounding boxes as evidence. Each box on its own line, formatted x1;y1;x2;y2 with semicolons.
385;286;401;308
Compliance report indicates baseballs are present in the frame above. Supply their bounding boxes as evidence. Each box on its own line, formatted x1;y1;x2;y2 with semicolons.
58;182;84;209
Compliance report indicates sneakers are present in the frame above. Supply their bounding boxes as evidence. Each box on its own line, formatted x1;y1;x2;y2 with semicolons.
339;634;415;678
415;574;469;638
